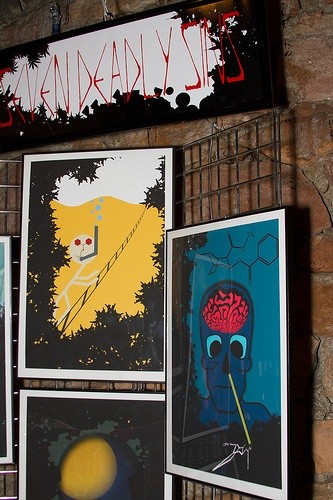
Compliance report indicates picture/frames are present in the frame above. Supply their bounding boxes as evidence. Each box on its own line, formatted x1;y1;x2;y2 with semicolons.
161;205;313;500
17;147;184;383
17;387;179;500
1;234;17;470
